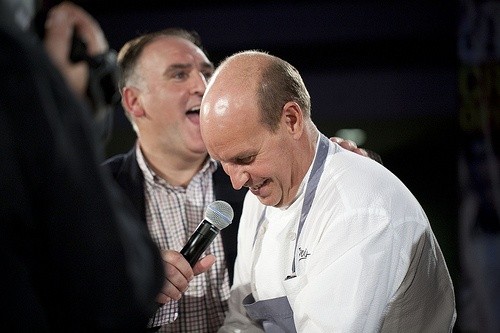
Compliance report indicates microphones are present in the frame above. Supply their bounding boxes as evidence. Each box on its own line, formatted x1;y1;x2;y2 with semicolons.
154;200;234;312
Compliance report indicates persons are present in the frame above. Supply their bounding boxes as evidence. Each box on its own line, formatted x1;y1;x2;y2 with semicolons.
200;49;458;333
98;28;368;333
45;2;136;160
0;0;164;332
